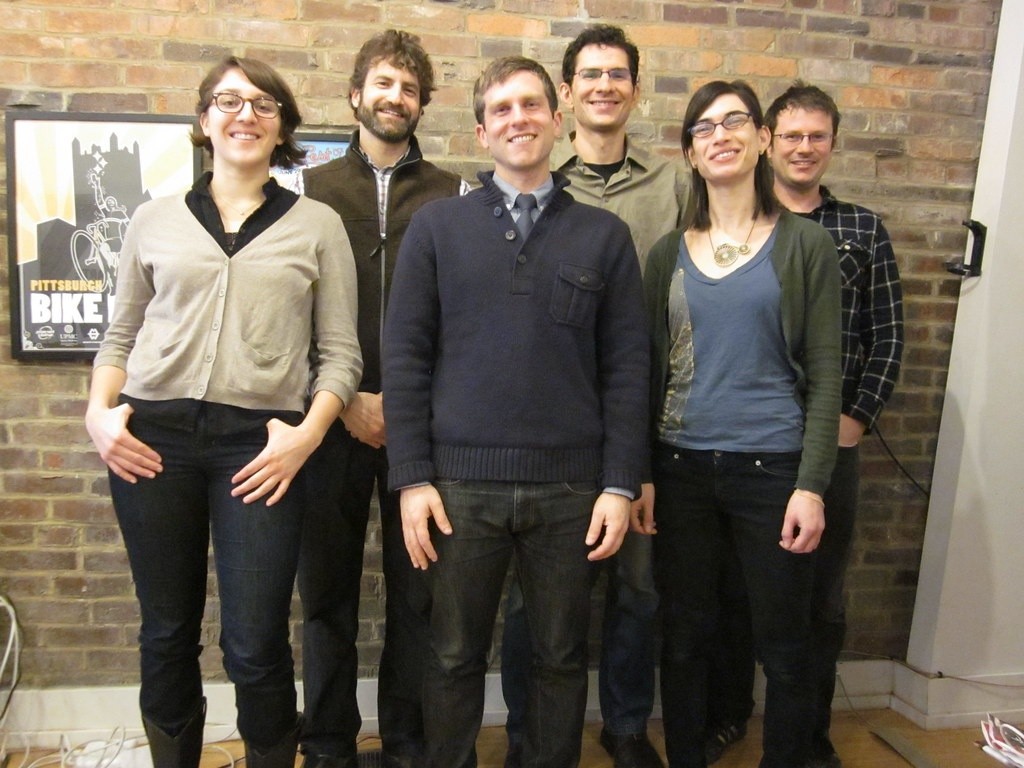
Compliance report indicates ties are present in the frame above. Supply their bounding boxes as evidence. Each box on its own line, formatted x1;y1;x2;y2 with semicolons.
513;193;538;243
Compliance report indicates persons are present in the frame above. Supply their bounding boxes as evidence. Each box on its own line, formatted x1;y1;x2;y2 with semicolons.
754;76;905;768
630;77;843;768
380;57;651;768
83;56;364;768
290;32;480;768
551;23;691;768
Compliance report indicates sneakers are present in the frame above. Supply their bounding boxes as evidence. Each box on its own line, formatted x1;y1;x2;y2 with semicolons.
800;727;842;768
701;713;746;765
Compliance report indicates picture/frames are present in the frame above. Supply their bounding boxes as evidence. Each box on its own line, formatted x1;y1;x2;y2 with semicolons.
5;111;203;363
263;133;354;198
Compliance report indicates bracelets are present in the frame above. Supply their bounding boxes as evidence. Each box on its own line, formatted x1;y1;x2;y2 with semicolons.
793;489;825;510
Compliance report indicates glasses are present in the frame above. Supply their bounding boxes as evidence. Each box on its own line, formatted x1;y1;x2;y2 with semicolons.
773;133;833;147
212;92;283;119
574;66;634;83
687;112;752;138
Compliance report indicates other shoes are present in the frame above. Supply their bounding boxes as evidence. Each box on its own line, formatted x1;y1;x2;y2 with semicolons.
301;748;358;768
382;747;431;768
599;724;663;768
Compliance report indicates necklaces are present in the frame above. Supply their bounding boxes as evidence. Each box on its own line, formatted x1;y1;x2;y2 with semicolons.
707;205;762;267
208;183;267;215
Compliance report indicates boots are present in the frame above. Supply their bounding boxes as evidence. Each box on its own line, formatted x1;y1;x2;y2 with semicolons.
245;713;305;768
142;696;207;767
